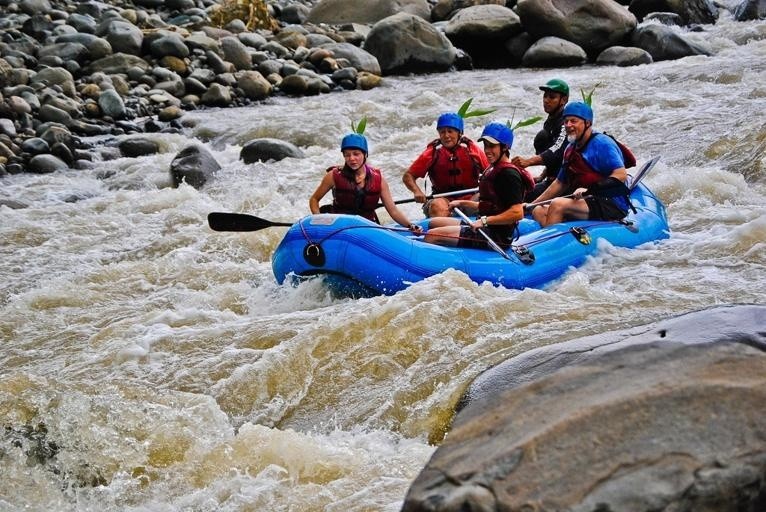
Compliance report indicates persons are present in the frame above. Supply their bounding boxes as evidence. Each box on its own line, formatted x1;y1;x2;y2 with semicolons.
511;79;572;204
424;123;536;251
403;114;487;216
310;133;422;236
524;102;639;228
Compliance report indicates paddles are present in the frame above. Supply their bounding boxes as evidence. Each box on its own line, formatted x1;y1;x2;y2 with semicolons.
524;156;662;212
319;187;480;214
207;212;295;232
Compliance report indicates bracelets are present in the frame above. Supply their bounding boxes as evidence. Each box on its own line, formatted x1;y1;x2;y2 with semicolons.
480;214;489;227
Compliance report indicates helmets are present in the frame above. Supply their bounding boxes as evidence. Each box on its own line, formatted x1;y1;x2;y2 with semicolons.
477;122;512;145
436;113;463;132
341;133;368;153
563;102;593;121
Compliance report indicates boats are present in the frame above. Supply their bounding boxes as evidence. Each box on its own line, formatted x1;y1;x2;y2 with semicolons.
272;171;673;299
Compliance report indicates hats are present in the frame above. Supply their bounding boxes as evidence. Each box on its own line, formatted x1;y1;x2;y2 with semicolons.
540;80;568;93
